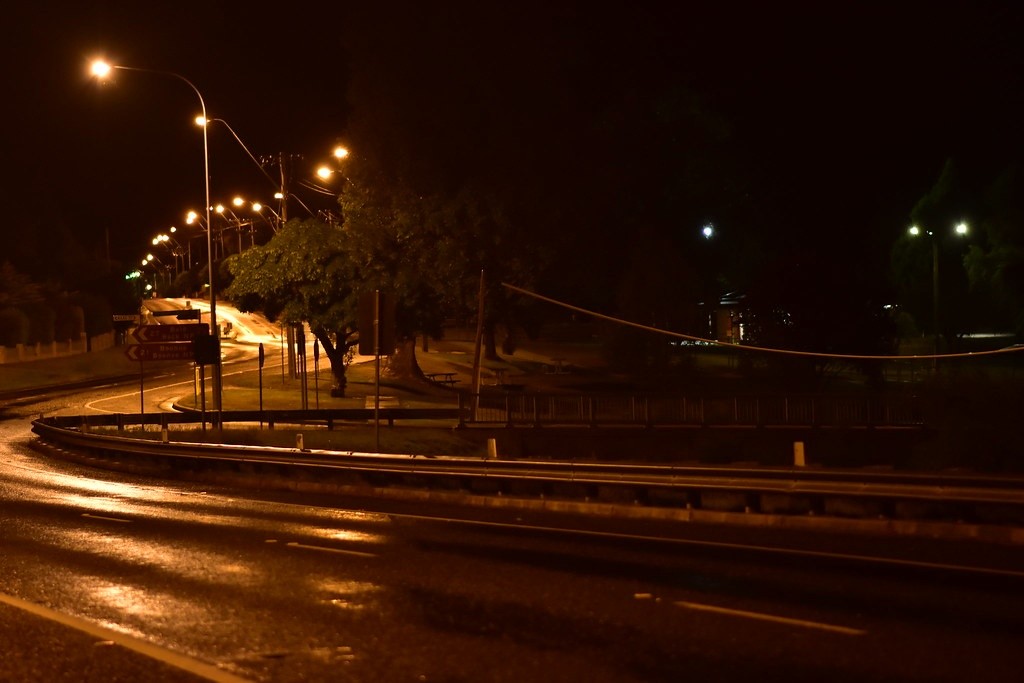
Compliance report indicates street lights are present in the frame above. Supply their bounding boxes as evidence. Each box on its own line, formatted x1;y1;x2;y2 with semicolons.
138;112;296;380
87;59;226;426
908;225;943;365
